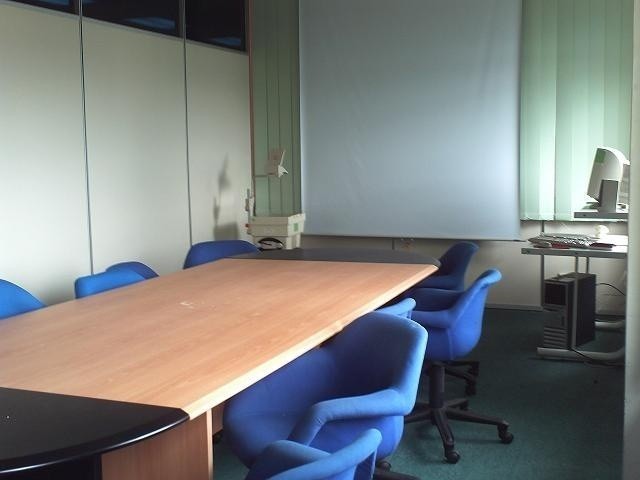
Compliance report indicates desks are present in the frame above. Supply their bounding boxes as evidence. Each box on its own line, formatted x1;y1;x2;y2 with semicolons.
1;247;442;478
521;233;628;363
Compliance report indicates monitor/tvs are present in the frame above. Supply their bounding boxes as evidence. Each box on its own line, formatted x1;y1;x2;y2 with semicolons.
587;147;630;205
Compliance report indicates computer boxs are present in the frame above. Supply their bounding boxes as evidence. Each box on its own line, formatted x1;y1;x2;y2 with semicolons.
543;272;596;350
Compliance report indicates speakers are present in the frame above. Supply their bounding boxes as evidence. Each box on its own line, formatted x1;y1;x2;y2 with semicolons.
598;179;618;212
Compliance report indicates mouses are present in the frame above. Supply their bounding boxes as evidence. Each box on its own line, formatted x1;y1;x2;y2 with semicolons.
533;241;552;248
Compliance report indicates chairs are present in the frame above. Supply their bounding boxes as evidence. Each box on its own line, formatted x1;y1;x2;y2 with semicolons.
371;297;417;320
404;269;514;464
1;279;46;320
410;241;481;397
241;428;383;480
222;311;430;480
182;241;261;270
106;260;160;281
73;268;147;300
